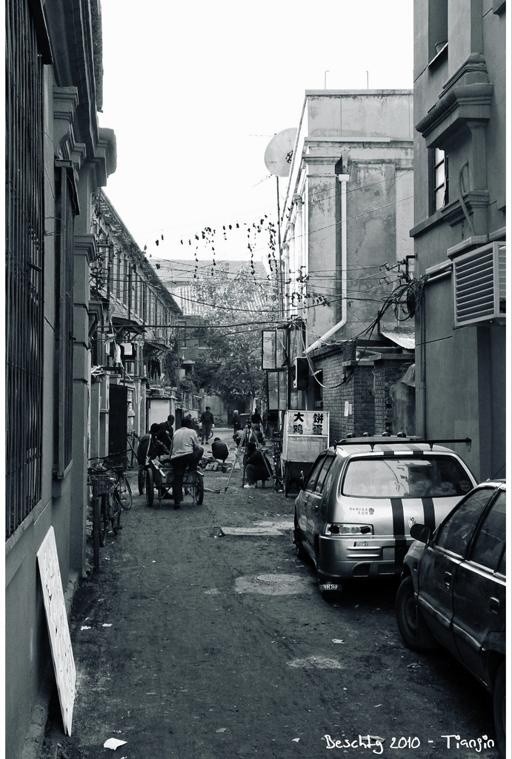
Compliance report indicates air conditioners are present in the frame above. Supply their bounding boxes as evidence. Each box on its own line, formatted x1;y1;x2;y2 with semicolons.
452;241;506;329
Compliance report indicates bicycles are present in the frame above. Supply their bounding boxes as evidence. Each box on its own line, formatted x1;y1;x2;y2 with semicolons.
87;445;133;567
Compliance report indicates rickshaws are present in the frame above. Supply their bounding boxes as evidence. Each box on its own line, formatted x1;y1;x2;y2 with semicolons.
145;454;205;505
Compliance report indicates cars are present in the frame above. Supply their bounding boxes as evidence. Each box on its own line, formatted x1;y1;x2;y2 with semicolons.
393;478;505;757
294;430;478;602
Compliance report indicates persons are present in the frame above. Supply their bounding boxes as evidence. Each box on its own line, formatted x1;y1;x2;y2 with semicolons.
136;422;167;491
199;405;215;446
156;423;173;500
169;417;204;509
229;406;267;448
240;442;274;489
211;436;230;465
164;414;174;440
